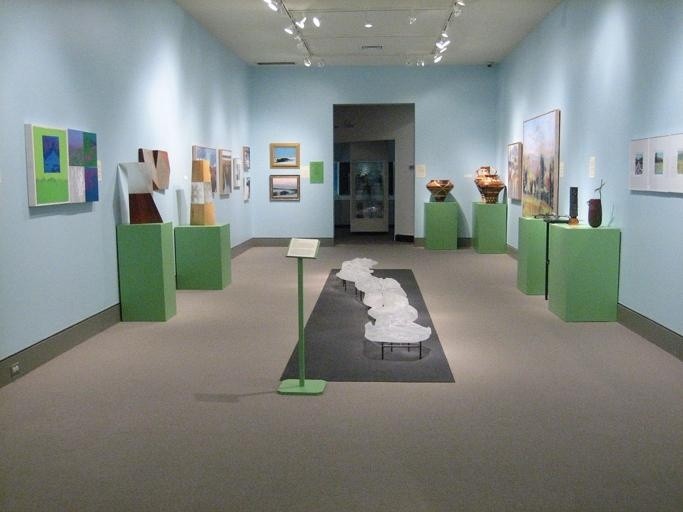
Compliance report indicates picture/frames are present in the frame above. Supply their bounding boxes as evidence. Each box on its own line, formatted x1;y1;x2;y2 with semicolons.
629;134;683;196
196;144;252;202
269;142;300;167
506;142;523;200
269;174;300;201
522;108;559;217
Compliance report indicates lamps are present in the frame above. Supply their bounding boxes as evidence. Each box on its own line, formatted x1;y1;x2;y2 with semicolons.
407;1;467;78
260;0;327;72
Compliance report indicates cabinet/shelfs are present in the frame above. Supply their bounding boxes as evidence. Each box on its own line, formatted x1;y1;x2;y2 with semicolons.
116;223;177;322
472;202;507;253
516;217;620;323
424;202;458;250
175;223;231;290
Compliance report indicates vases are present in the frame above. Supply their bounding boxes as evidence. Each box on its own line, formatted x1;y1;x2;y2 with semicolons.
474;166;504;203
588;198;602;227
568;186;581;224
426;179;454;201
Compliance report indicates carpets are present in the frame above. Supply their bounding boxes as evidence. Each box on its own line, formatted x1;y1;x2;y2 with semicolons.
279;268;457;384
